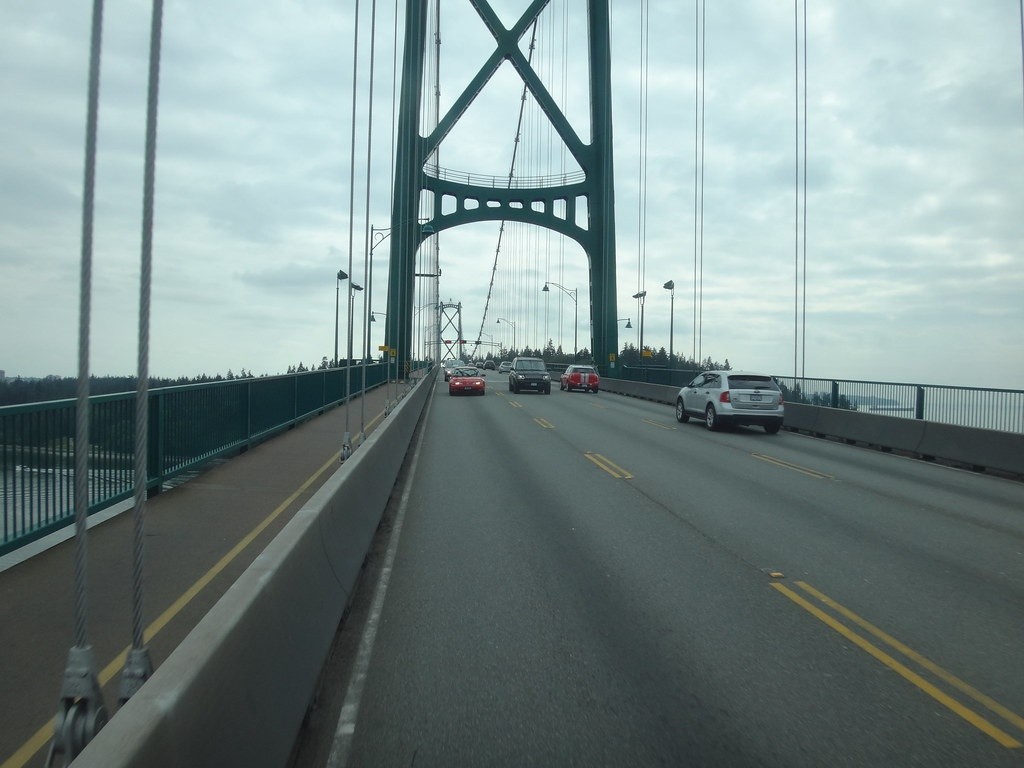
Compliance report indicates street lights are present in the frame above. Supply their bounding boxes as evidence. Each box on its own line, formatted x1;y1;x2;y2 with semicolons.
349;280;363;362
366;216;436;364
632;291;648;361
496;317;516;352
332;269;348;369
542;281;579;364
663;279;675;356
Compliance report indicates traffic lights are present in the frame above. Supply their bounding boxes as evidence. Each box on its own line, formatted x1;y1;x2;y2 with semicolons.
476;341;482;344
460;340;467;343
446;340;452;344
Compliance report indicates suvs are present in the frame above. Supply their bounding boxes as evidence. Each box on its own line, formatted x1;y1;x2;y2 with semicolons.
508;357;551;394
675;370;785;434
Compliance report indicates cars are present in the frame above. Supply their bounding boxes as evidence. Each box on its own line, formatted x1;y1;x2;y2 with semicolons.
475;361;483;368
467;363;475;367
440;358;468;382
483;359;496;370
449;366;486;396
498;361;511;374
559;365;599;393
